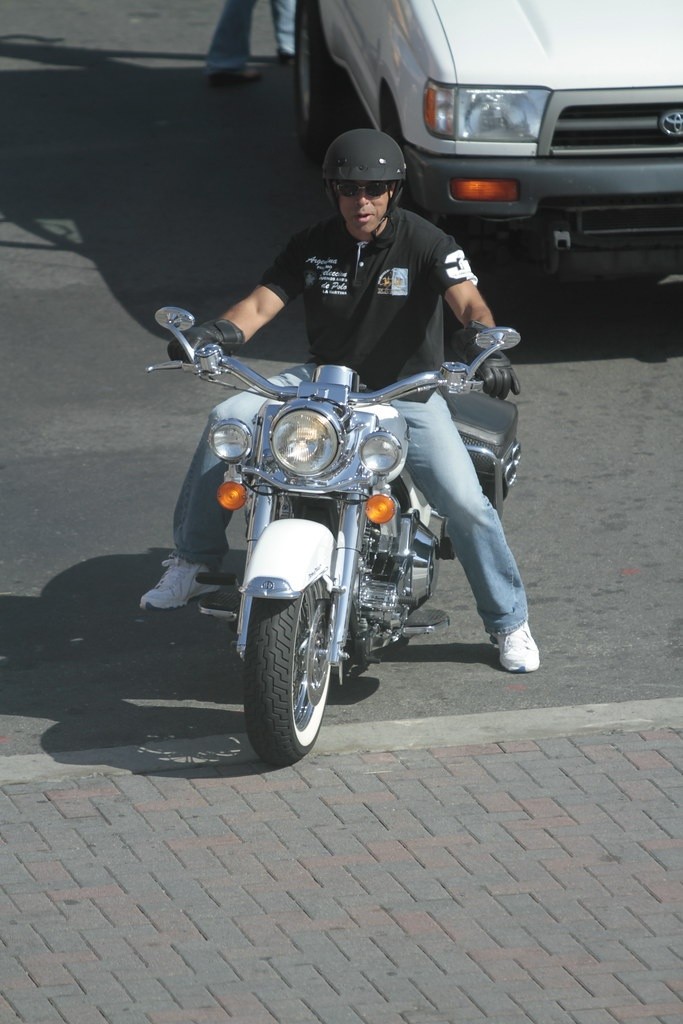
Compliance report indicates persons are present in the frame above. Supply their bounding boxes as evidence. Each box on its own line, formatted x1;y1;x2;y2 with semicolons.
204;0;295;89
140;129;541;675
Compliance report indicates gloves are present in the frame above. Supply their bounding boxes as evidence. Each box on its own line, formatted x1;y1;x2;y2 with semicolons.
168;319;245;365
463;321;521;400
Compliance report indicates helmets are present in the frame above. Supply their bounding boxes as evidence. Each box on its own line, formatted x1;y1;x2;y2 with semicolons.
323;129;406;212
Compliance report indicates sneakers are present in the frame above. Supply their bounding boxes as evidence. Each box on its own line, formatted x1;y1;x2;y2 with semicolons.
139;555;220;612
492;621;540;673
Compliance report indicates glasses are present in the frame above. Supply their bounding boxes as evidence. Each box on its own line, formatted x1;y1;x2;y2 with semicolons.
334;180;393;197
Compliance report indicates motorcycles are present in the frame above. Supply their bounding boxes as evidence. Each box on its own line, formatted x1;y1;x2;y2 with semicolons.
141;305;521;765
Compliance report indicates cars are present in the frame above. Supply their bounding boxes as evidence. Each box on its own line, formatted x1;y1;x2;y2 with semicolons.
292;2;683;305
